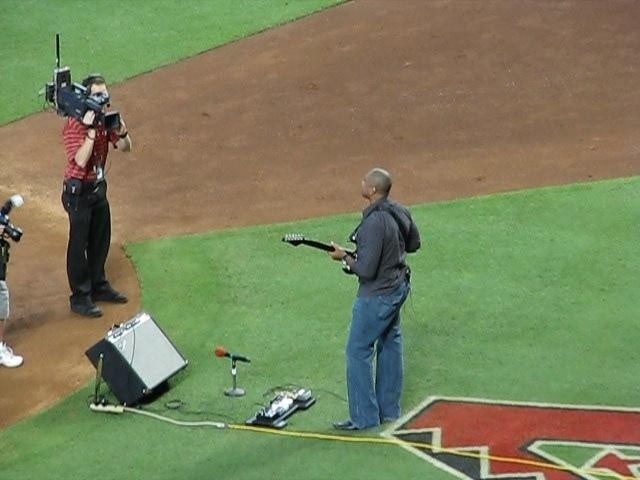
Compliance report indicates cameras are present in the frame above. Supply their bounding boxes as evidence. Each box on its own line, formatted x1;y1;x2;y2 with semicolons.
0;194;25;243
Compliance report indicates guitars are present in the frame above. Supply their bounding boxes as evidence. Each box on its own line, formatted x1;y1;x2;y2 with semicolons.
282;234;410;286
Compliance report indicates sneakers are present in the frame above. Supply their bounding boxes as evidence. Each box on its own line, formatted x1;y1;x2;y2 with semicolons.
0;342;24;369
92;285;128;303
70;296;103;317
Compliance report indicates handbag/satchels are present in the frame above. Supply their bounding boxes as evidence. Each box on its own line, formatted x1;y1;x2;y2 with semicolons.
62;180;107;206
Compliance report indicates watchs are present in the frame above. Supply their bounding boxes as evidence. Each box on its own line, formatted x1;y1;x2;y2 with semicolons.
342;254;349;265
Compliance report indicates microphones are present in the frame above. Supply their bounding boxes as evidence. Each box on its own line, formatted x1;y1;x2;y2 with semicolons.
214;346;251;363
106;104;110;107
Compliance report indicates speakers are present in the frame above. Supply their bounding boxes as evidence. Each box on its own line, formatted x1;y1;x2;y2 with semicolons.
84;311;189;405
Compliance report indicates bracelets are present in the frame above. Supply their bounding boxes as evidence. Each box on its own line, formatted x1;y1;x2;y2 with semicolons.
117;131;128;138
86;133;95;140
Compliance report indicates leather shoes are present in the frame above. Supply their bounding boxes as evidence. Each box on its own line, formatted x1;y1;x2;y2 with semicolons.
332;421;357;430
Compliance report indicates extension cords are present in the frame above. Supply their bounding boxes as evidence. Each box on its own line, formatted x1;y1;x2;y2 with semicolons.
89;402;124;413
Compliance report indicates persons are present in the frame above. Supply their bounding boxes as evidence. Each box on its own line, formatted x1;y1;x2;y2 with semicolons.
327;168;421;429
0;224;24;367
61;76;131;318
